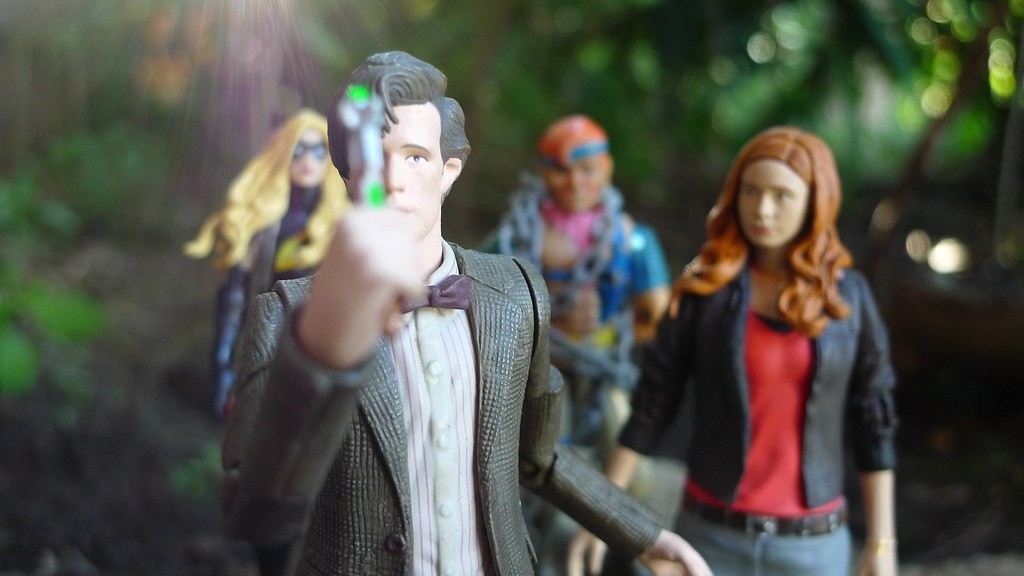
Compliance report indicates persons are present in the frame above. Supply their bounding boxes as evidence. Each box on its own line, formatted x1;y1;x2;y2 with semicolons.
189;107;350;418
220;44;716;574
474;115;671;575
566;126;905;576
